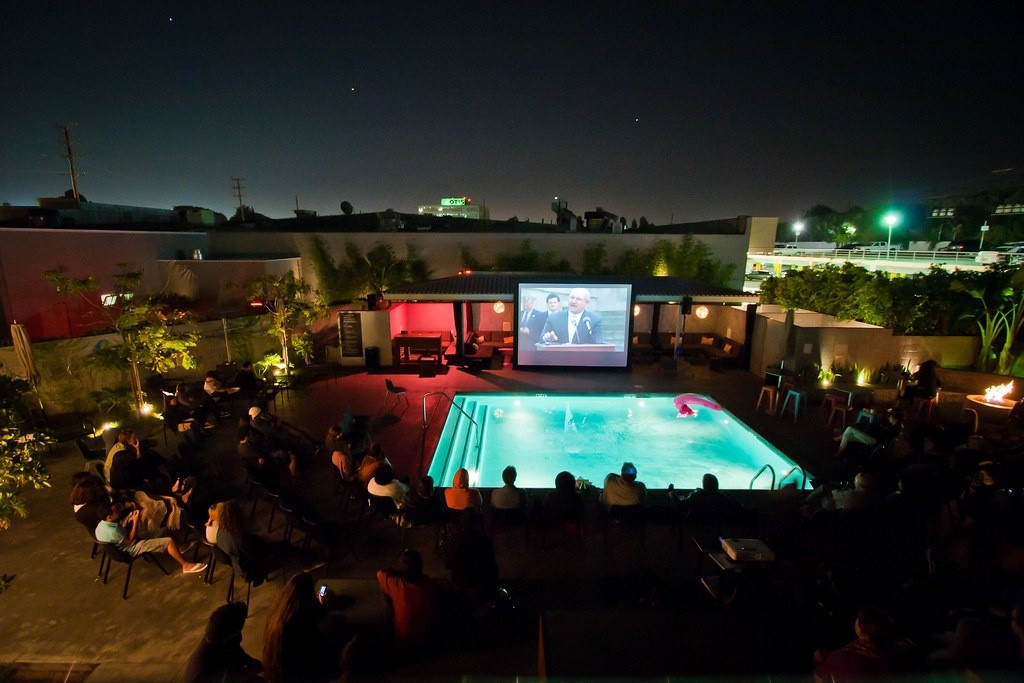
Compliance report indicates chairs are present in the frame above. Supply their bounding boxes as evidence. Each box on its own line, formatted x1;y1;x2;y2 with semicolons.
74;379;1024;619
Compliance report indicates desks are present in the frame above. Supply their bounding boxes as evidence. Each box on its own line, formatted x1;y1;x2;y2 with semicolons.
763;367;798;416
829;385;872;407
393;334;443;370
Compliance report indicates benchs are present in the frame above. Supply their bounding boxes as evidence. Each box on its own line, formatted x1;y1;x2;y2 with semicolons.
464;330;744;377
401;330;457;364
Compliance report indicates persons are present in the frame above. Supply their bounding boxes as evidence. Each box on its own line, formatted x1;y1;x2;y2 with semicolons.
544;472;584;508
69;426;297;575
184;602;266;683
897;360;941;400
325;424;483;530
263;573;331;683
519;295;543;351
378;549;431;647
833;410;899;452
812;598;895;683
906;414;972;460
165;395;215;436
540;293;560;336
668;474;718;508
540;288;603;344
329;635;369;683
491;467;527;509
235;361;259;395
603;462;648;508
204;369;231;417
791;468;871;513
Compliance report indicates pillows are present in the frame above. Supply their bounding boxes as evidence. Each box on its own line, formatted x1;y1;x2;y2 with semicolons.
475;335;485;343
470;342;479;352
502;336;514;343
723;343;733;354
632;336;638;344
670;336;682;344
700;336;715;346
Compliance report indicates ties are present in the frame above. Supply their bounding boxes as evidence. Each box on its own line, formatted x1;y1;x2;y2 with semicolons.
521;311;528;327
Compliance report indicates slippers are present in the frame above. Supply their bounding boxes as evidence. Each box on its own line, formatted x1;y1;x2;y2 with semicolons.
180;540;197;554
183;563;208;573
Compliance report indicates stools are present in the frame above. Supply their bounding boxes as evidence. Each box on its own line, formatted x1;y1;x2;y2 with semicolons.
779;383;799;416
419;357;436;377
826;407;847;428
757;385;776;416
821;393;846;418
855;410;880;425
908;397;935;426
780;389;807;422
